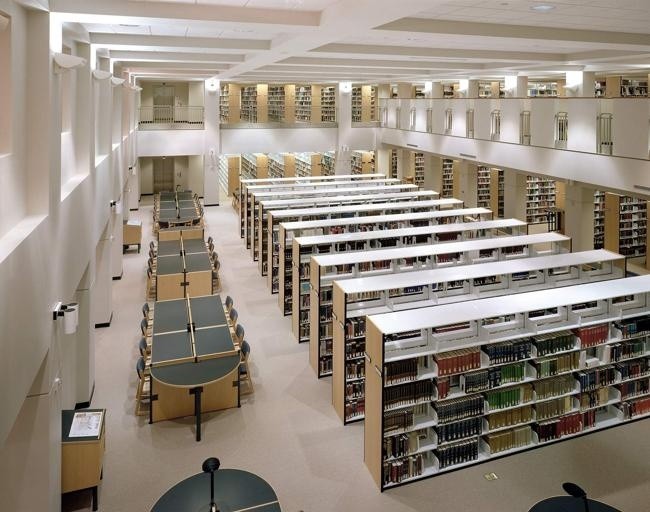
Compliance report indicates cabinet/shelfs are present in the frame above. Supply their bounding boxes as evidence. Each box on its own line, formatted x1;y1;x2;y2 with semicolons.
220;146;650;494
378;75;650;100
219;76;377;124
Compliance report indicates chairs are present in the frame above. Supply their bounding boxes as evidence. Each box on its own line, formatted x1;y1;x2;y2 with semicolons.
135;304;153;416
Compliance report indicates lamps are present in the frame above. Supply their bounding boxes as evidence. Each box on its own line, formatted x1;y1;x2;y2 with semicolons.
92;70;144;91
562;482;589;512
53;301;79;334
111;200;122;214
55;52;86;74
209;83;216;91
128;160;136;176
202;458;221;512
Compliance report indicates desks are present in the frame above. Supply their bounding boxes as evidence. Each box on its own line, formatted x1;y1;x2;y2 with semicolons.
148;190;242;442
61;409;106;509
148;468;281;512
123;218;142;253
526;496;623;512
